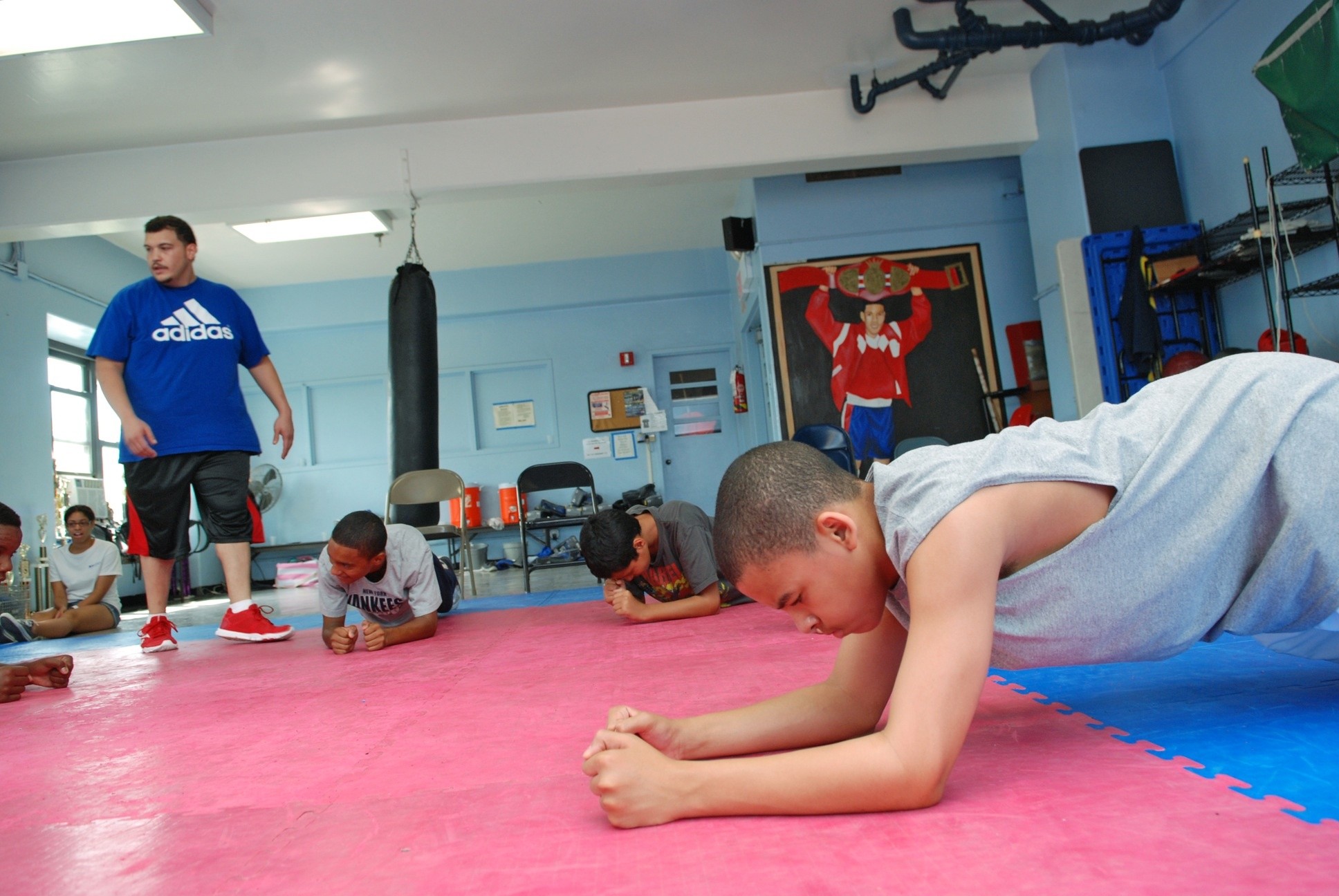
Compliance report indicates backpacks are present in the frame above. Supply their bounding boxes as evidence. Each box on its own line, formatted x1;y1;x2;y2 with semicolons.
1256;327;1309;356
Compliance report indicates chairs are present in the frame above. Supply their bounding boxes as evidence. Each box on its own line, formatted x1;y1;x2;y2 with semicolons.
791;423;858;477
894;435;949;461
515;460;603;593
383;468;476;603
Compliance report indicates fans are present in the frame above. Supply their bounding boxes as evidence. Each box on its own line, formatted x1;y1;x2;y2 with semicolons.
246;463;283;514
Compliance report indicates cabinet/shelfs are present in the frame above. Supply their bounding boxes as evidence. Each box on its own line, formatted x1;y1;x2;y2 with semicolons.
1128;146;1339;382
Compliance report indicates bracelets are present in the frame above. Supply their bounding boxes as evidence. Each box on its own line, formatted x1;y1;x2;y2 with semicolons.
71;605;79;609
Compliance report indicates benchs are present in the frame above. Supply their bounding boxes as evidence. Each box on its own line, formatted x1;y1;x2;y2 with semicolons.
247;517;550;589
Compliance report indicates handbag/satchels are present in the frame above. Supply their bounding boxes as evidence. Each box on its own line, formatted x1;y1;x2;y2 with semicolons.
613;500;627;511
584;492;603;505
570;487;589;507
622;483;656;508
539;499;566;518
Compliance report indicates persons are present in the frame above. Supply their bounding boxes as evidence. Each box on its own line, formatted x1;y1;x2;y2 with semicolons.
85;216;295;653
580;500;757;623
581;352;1339;829
805;263;932;477
0;505;123;645
319;510;462;655
0;503;73;703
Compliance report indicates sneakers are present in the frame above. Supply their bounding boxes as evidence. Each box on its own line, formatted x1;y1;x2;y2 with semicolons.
139;616;178;653
214;604;295;643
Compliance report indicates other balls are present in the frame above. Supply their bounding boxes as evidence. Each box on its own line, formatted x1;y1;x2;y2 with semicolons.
1161;350;1209;375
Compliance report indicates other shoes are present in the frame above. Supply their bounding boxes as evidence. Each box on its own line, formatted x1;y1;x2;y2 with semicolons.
0;611;44;644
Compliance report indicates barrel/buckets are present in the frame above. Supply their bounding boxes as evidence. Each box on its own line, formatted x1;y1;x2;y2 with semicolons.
447;482;484;528
463;543;489;570
498;482;527;524
503;541;527;566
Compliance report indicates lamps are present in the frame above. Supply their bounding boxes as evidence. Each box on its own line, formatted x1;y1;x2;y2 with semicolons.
227;209;392;245
0;0;213;58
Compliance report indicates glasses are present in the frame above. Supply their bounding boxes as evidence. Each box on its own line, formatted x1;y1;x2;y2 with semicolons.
64;519;91;528
